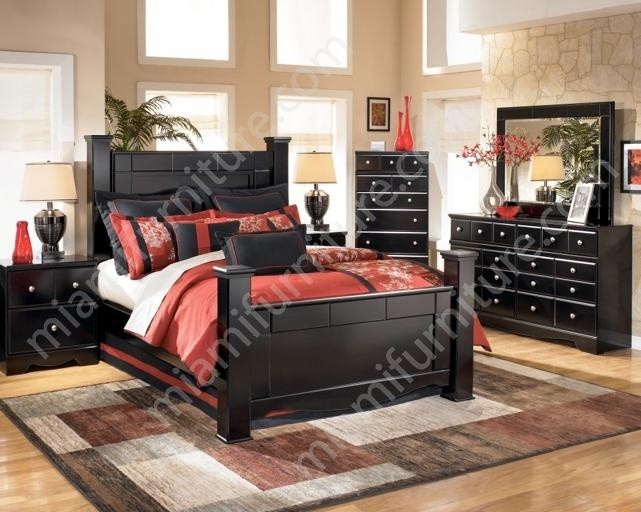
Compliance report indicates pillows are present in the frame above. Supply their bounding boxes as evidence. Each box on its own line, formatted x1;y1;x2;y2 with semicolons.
94;182;321;280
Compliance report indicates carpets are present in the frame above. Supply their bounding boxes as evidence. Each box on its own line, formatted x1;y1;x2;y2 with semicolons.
0;350;641;512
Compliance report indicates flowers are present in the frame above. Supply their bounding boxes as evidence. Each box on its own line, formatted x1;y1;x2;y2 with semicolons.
456;123;504;167
504;128;541;166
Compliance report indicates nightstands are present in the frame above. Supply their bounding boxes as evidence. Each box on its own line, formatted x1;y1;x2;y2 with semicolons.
302;230;348;246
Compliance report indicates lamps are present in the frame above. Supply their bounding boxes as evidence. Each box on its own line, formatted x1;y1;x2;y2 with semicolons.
18;160;78;260
293;150;338;232
529;154;566;202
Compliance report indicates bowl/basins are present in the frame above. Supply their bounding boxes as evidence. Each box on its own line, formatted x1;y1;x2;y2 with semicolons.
496;206;520;218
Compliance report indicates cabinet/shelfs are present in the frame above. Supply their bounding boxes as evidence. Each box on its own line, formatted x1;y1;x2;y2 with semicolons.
0;254;100;376
355;151;429;267
448;214;633;355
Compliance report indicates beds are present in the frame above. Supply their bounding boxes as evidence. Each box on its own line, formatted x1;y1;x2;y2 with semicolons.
84;135;479;445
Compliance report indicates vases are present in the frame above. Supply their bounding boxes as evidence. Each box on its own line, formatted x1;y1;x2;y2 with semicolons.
510;164;518;202
13;220;34;265
394;112;405;151
402;96;414;151
480;166;506;214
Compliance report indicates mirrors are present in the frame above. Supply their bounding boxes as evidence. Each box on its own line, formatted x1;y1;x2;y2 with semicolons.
496;101;615;226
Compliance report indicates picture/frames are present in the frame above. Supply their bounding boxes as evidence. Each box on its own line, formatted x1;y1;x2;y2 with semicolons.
567;184;595;224
620;140;641;193
367;97;390;131
